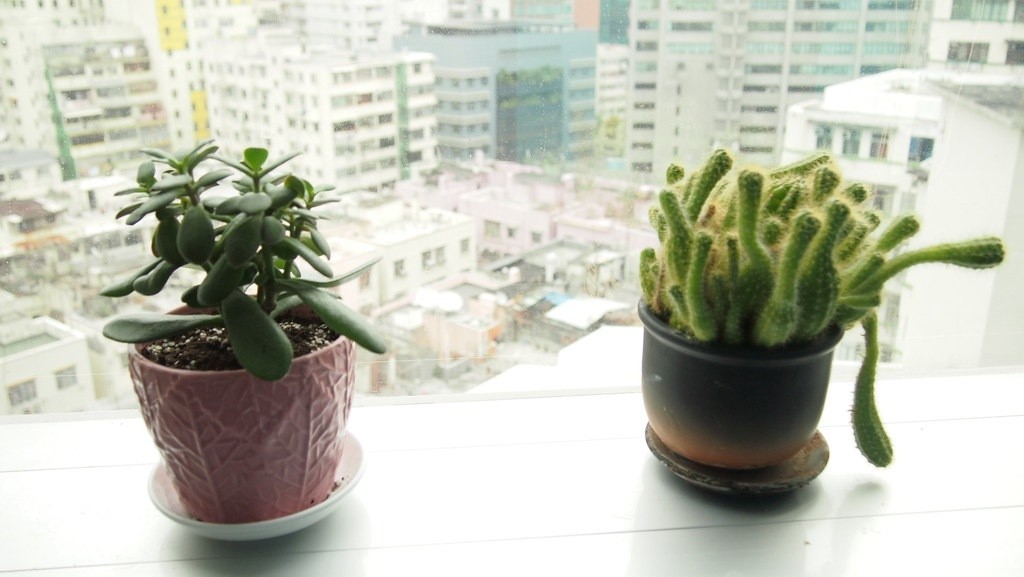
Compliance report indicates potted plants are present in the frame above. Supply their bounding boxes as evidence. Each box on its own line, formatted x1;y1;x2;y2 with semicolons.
102;140;387;523
638;147;1003;469
